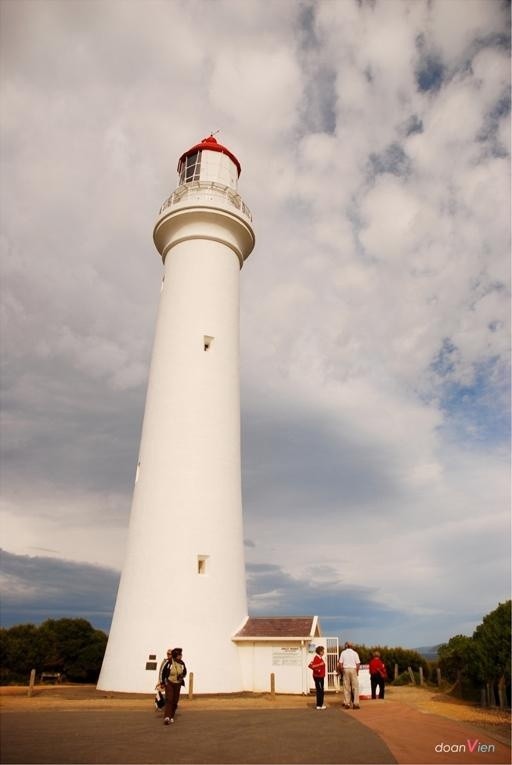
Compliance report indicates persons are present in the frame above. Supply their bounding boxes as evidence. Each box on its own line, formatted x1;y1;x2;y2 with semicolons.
339;642;354;707
338;641;361;709
308;646;327;710
159;649;173;676
160;647;187;725
369;651;386;701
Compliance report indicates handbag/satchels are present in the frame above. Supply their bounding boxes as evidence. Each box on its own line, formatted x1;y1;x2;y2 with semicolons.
308;661;321;673
155;691;165;708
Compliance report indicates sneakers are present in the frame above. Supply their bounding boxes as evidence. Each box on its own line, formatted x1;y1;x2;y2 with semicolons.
343;703;360;709
317;705;326;710
165;717;174;724
